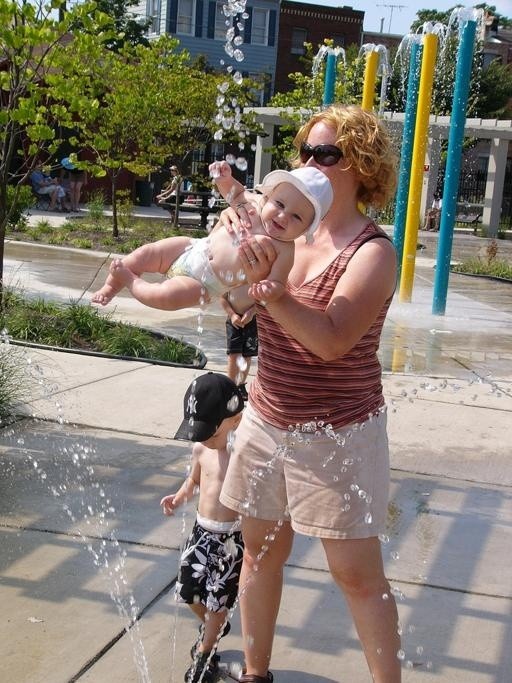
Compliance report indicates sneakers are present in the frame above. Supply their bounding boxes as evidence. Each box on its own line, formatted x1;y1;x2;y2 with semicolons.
183;651;221;682
189;618;232;658
235;381;248;400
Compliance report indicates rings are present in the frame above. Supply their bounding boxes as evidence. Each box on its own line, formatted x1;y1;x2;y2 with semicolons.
236;203;244;210
248;258;257;265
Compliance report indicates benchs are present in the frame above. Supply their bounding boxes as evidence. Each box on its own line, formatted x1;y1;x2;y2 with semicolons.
432;202;503;235
160;190;219;229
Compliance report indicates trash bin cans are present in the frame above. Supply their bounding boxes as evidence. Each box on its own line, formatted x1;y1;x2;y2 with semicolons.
135;179;155;207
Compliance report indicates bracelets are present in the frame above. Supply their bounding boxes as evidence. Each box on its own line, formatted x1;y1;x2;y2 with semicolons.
260;298;270;307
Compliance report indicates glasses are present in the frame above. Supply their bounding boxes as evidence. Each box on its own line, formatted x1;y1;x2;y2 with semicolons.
295;141;345;166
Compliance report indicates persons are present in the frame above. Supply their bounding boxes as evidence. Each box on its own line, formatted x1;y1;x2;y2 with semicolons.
158;372;247;682
42;166;63;185
30;158;68;211
419;190;443;231
92;159;334;310
207;109;401;681
156;164;184;221
220;282;257;398
58;138;89;212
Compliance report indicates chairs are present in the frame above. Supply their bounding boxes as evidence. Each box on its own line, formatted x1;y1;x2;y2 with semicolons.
28;176;61;211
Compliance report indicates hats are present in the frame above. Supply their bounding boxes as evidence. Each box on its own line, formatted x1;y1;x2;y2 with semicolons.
173;371;244;442
253;167;334;246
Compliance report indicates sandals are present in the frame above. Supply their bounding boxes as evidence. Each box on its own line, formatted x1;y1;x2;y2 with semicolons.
239;669;273;682
